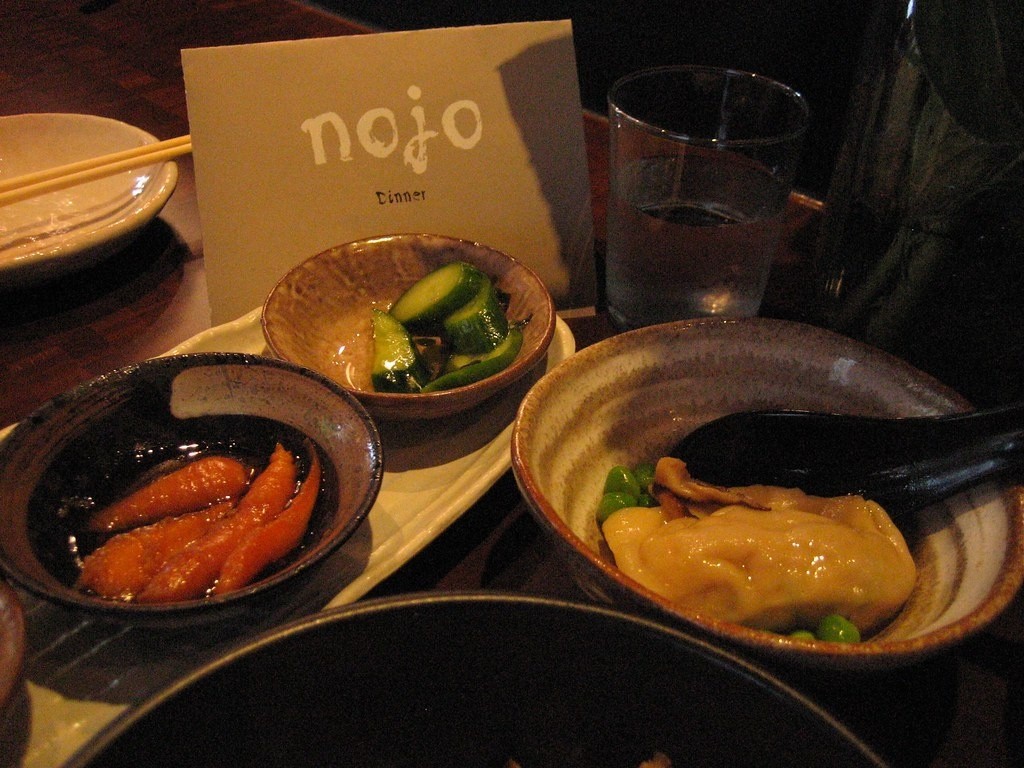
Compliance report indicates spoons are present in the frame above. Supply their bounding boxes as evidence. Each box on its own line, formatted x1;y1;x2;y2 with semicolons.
666;398;1024;523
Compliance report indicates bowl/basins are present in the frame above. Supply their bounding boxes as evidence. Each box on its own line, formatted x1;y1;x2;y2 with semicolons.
58;587;893;768
0;351;386;634
259;231;557;426
0;112;180;299
510;316;1023;673
0;574;28;718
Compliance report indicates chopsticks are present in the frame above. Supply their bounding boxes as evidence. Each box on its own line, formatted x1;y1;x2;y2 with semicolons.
0;132;194;207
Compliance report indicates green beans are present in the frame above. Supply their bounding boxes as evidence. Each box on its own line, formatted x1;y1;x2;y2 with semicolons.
596;461;660;524
759;614;861;643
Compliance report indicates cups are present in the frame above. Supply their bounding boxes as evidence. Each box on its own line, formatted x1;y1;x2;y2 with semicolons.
601;63;814;333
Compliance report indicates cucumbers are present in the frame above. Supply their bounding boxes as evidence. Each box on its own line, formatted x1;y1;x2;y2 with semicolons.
371;259;523;392
